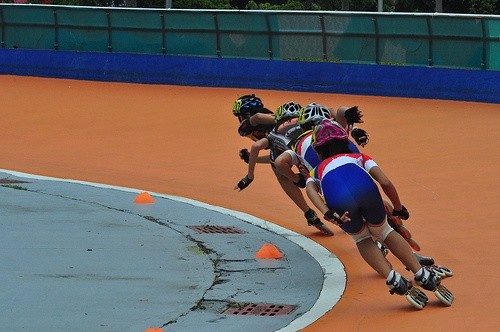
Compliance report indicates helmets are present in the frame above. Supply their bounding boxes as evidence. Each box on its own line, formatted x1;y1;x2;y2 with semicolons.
298;103;331;126
275;102;303;121
311;119;350;148
232;93;264;116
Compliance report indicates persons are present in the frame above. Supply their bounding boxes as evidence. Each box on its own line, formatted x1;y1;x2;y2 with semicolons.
274;102;435;267
232;92;370;236
306;118;442;296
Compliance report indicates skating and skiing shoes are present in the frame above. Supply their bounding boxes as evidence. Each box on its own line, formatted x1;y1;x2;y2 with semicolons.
386;270;429;311
412;252;453;279
414;268;454;307
304;208;334;236
375;240;388;257
380;199;422;252
